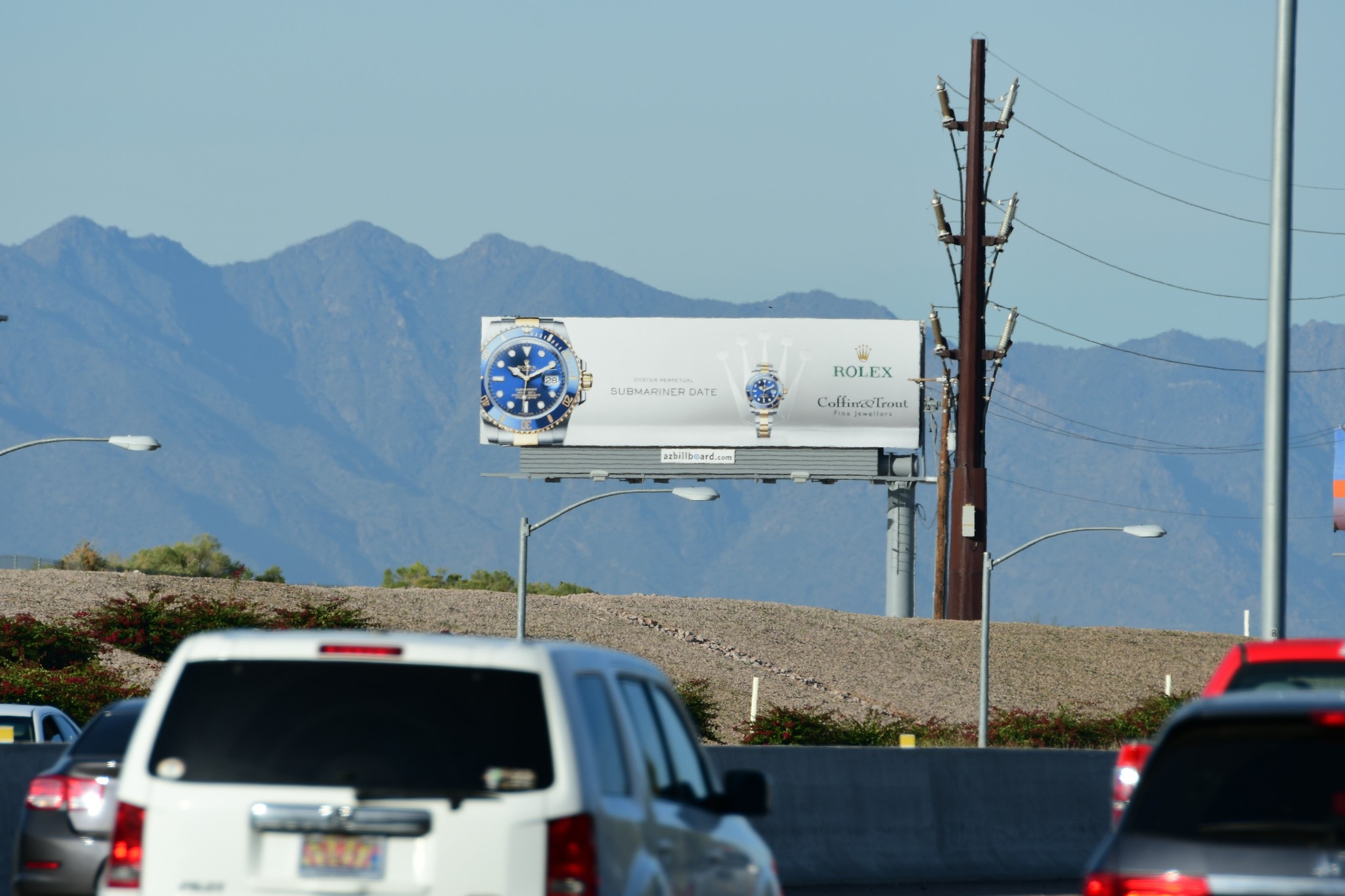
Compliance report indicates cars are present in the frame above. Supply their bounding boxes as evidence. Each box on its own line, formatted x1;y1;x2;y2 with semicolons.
1080;638;1344;896
0;697;147;896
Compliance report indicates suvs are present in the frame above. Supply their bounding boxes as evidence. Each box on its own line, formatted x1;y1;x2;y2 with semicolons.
98;631;784;896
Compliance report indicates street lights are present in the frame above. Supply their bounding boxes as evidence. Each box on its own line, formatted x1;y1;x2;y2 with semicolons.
978;523;1167;747
517;485;720;642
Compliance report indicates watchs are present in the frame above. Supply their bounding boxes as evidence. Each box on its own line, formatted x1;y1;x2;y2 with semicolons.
481;315;592;447
745;362;788;438
